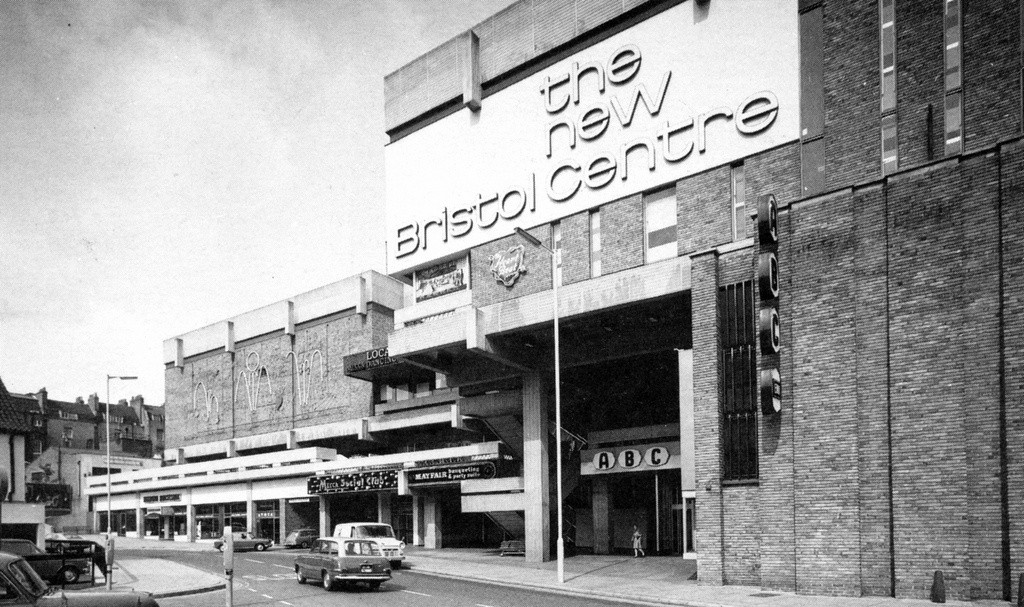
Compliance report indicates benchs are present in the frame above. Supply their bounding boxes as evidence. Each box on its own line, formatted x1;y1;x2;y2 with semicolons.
498;540;526;558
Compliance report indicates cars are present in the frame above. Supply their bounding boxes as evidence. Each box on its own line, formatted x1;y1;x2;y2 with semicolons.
283;528;319;549
214;531;274;552
0;537;90;585
293;535;393;592
0;550;159;607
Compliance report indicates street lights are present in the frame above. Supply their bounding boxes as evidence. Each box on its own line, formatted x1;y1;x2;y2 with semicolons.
103;375;138;589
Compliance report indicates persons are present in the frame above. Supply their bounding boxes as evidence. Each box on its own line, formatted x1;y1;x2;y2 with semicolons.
630;524;645;558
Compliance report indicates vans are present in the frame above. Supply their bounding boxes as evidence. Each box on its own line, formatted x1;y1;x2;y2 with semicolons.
332;521;406;569
